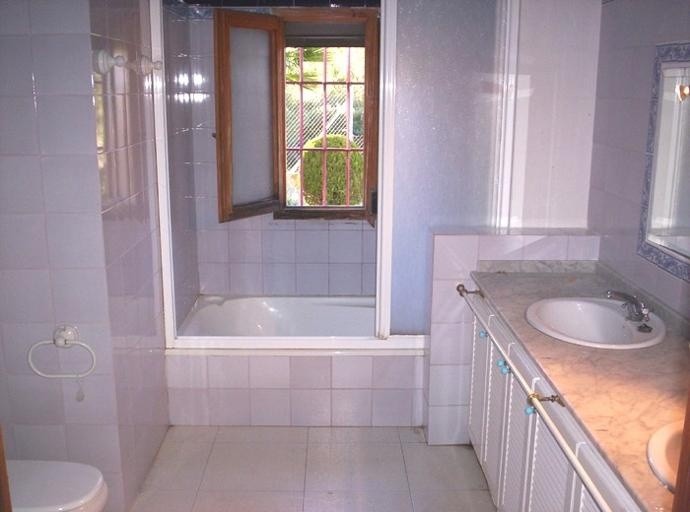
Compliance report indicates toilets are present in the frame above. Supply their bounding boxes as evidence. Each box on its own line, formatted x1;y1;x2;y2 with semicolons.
3;460;110;512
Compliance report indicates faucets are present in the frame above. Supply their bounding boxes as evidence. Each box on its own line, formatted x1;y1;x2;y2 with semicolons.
605;289;644;322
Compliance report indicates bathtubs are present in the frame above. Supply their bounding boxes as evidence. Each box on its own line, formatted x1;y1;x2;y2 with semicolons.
176;293;376;336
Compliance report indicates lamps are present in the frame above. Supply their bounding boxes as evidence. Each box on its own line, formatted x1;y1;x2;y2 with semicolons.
94;49;125;74
141;54;162;76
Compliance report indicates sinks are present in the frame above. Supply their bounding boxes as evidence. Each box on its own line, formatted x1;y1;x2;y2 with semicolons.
525;296;666;350
646;420;686;493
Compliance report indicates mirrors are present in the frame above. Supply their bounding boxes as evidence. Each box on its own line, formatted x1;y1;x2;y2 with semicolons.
637;41;690;284
